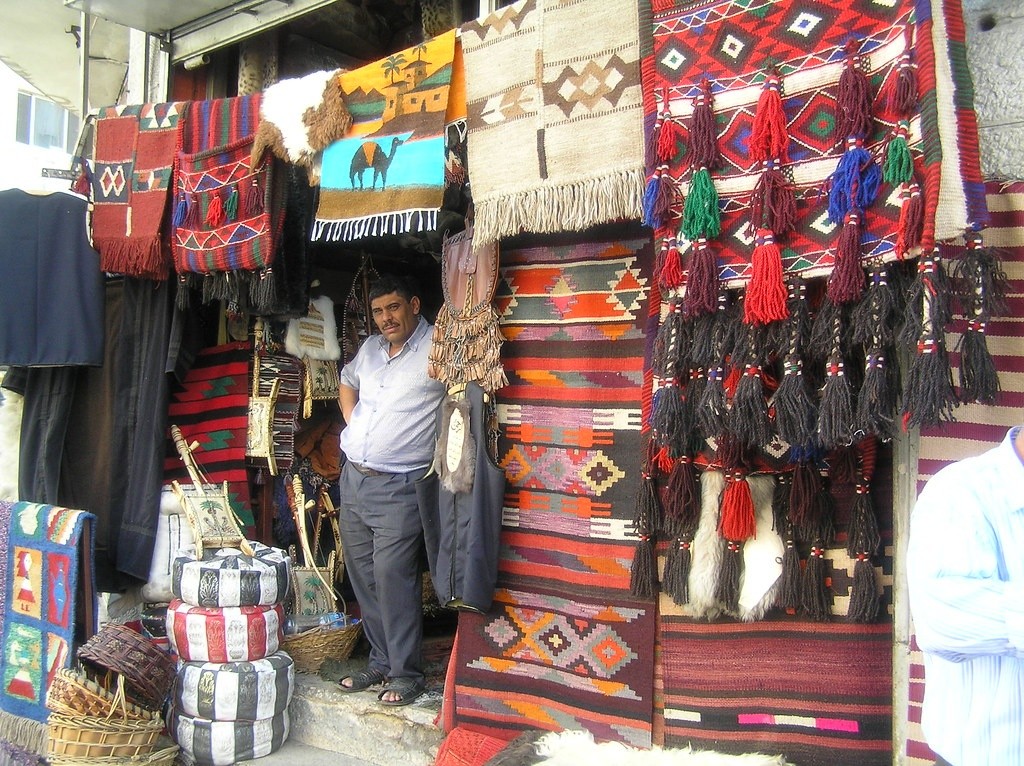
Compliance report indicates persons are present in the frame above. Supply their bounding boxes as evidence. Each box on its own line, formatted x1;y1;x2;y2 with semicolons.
905;427;1024;766
337;269;449;706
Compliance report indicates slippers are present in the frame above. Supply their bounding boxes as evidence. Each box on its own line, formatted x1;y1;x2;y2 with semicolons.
378;678;425;705
335;663;391;693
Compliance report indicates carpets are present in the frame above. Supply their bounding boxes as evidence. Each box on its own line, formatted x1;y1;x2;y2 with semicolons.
0;0;1024;765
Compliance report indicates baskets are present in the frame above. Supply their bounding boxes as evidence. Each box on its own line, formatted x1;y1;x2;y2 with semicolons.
45;623;180;766
279;614;364;676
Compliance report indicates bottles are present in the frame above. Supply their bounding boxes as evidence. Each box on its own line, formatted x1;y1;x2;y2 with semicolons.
283;612;358;636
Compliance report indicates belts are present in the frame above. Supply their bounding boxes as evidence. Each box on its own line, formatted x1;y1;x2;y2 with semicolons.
351;462;382;476
